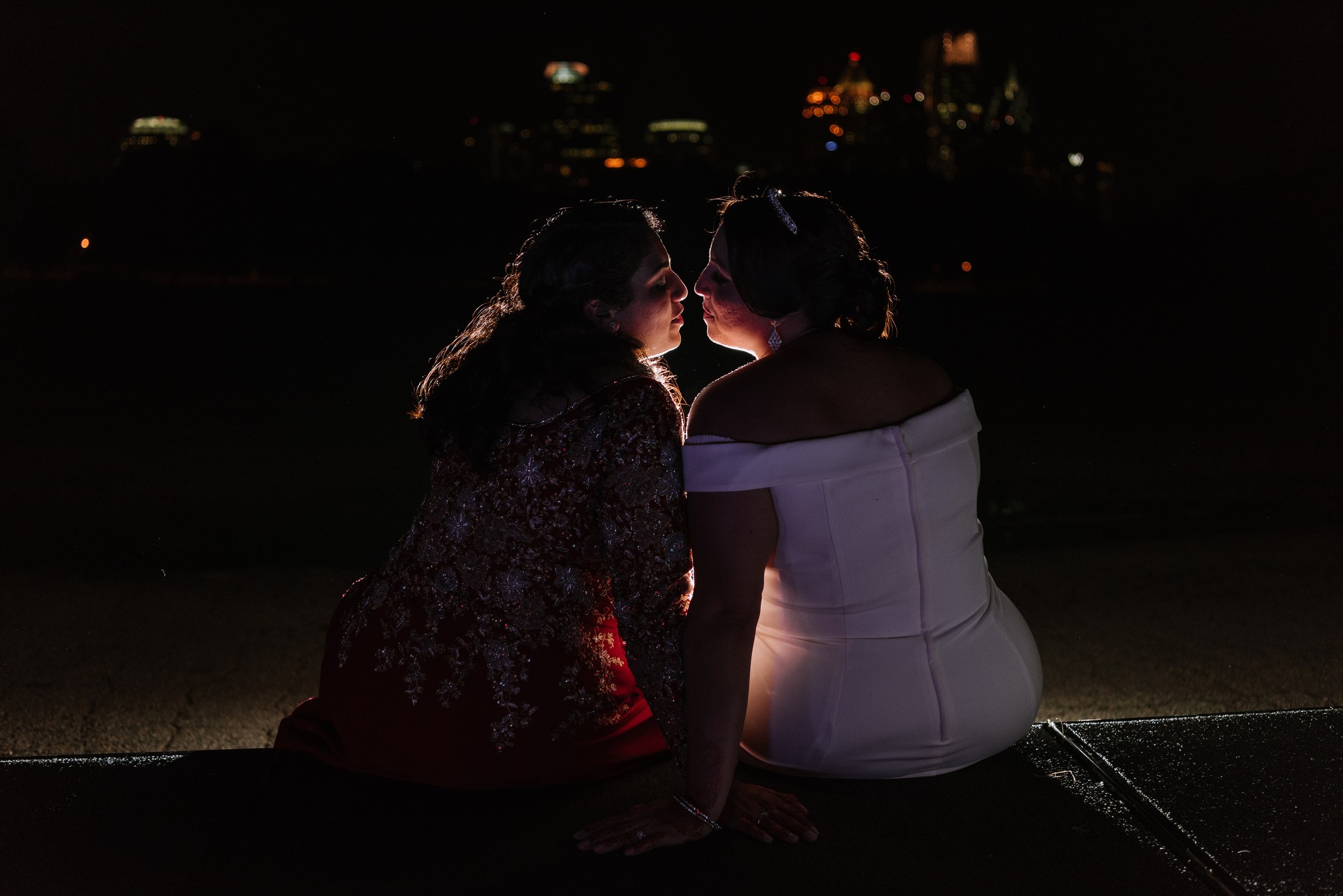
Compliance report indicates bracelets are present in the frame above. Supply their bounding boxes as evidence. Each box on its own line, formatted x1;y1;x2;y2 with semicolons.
673;794;724;833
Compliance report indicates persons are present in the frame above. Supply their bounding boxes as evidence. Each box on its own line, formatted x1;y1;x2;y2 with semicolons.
572;179;1045;857
264;193;821;849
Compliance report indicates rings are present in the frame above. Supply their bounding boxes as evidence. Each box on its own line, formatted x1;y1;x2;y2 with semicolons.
634;826;647;841
757;811;768;828
